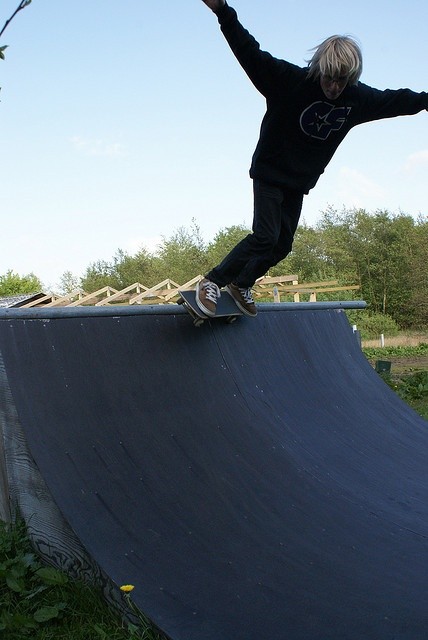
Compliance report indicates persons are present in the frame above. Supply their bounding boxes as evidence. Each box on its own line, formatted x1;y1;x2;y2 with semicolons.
191;0;428;317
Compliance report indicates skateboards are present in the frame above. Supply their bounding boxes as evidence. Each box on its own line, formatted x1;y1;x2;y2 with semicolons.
176;290;244;328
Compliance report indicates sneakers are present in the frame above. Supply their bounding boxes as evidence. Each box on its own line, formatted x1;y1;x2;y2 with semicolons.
195;278;221;317
225;282;258;318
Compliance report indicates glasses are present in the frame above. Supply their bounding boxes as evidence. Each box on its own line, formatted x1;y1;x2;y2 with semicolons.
320;74;348;86
